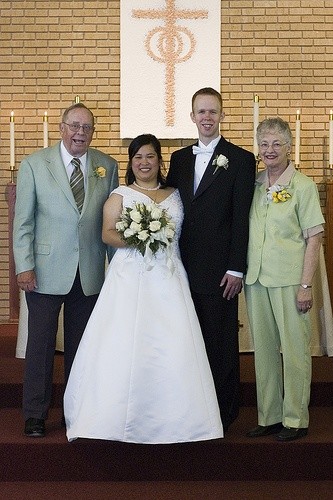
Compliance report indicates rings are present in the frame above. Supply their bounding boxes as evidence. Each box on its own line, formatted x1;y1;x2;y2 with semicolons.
309;305;311;308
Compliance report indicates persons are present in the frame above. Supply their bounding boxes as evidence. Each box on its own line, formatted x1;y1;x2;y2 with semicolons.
242;119;333;439
11;105;120;438
163;88;257;433
64;136;224;443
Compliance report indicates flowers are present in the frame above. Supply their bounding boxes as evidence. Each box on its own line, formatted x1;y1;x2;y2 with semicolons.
266;184;291;203
88;166;107;179
113;199;176;276
212;154;229;175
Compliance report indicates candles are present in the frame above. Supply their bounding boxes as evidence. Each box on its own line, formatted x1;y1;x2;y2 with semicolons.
43;112;48;148
10;112;15;166
295;110;300;164
329;110;333;164
254;93;259;154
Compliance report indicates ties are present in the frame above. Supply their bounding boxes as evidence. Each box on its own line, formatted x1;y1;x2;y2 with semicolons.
70;158;84;214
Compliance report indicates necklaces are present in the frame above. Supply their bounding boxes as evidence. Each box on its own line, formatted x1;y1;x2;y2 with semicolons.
134;181;162;192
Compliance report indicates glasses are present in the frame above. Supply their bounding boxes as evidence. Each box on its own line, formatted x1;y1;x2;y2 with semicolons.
62;121;94;133
258;142;288;150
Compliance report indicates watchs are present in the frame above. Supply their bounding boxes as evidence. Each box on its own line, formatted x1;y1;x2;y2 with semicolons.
299;283;311;289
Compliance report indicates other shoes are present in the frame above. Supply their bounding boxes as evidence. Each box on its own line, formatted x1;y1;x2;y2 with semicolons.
274;426;308;441
246;422;283;436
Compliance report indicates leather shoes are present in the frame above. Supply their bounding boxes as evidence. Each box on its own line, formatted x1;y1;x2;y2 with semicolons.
24;418;45;437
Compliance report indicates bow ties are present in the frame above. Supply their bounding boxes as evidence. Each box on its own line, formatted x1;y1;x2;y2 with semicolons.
192;146;212;154
